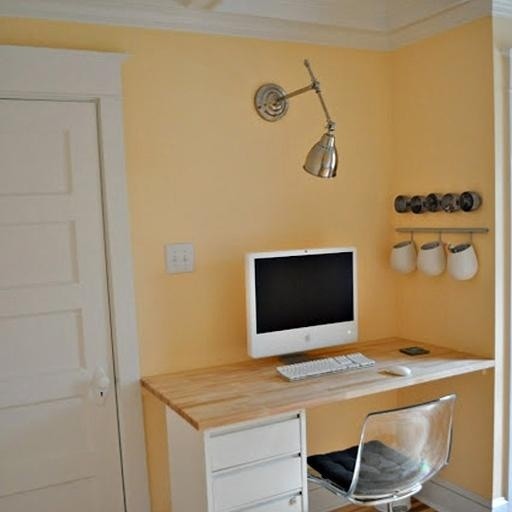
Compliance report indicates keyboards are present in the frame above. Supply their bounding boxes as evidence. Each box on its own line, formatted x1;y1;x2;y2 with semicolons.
274;351;375;382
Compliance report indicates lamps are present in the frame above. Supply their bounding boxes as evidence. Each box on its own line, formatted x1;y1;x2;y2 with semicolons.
251;59;341;181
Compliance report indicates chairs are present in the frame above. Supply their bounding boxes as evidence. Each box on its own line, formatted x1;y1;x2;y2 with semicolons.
306;392;460;512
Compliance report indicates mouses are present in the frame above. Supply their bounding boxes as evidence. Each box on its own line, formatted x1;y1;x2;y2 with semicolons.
380;364;410;377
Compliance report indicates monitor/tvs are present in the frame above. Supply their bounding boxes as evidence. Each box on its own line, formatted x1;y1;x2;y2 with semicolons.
245;246;359;359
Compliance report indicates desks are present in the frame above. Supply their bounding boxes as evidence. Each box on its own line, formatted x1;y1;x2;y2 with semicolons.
139;334;493;511
